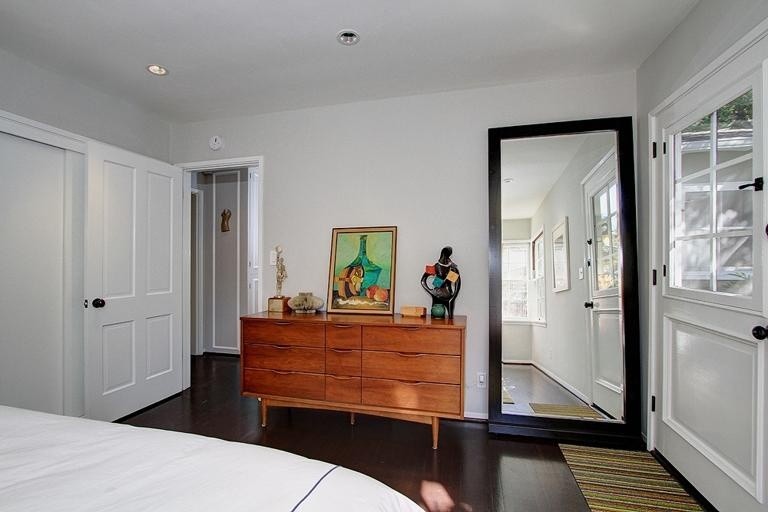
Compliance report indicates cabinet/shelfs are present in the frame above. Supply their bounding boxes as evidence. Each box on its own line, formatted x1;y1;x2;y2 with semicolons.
240;310;468;451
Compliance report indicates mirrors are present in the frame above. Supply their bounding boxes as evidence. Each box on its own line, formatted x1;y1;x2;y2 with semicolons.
486;115;641;443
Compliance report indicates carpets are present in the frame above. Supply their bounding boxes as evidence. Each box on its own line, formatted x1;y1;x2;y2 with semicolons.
528;402;606;419
558;443;705;512
503;387;515;405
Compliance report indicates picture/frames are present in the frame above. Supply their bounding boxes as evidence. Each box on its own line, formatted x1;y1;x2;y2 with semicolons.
326;225;397;316
551;216;571;293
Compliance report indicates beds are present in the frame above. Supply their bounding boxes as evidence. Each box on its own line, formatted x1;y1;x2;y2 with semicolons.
0;404;423;511
